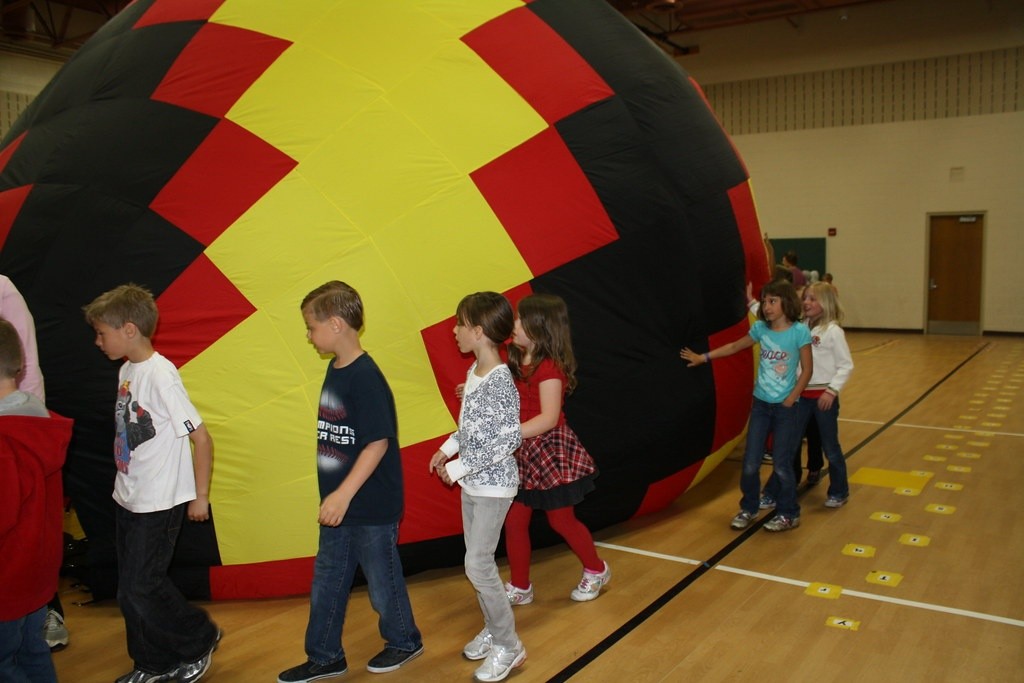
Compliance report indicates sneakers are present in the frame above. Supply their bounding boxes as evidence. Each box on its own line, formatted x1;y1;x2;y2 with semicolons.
762;451;773;465
114;625;223;683
277;656;348;683
759;495;776;509
729;510;759;530
463;627;492;660
366;642;424;673
824;496;848;508
43;609;68;653
504;580;533;606
763;515;800;532
570;560;612;602
475;633;529;683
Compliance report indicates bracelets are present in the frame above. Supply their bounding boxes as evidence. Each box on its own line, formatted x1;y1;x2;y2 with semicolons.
704;353;710;363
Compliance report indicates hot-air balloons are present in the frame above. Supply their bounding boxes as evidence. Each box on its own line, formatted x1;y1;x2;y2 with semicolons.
0;2;772;603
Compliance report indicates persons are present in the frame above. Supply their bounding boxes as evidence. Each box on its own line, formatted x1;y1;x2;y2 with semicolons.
680;280;813;530
456;294;610;606
797;282;854;507
83;285;224;683
0;277;74;683
430;292;528;682
758;250;846;509
277;280;424;683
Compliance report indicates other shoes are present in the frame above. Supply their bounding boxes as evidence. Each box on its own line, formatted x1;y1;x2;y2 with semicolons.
800;478;820;488
